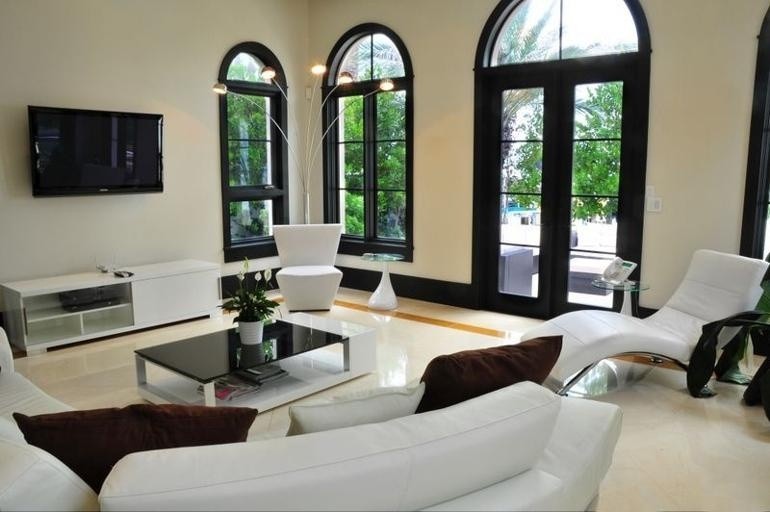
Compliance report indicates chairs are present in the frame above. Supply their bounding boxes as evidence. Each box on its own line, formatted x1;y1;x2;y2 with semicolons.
519;249;770;398
272;223;343;313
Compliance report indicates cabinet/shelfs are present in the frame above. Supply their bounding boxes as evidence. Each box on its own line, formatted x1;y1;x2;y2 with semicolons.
0;260;221;358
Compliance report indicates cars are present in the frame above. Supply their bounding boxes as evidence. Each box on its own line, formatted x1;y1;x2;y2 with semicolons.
501;200;541;218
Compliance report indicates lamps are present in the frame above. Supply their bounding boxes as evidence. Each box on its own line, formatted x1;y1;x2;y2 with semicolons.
212;61;395;224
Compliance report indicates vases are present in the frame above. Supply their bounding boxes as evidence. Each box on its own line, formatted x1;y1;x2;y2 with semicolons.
237;319;264;345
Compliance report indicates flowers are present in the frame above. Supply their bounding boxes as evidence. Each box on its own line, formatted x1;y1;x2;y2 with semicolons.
216;257;282;323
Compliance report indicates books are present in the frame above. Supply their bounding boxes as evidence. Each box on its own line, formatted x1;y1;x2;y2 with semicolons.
196;363;289;402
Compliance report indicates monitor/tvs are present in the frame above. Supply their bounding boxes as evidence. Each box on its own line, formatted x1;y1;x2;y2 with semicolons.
27;103;164;199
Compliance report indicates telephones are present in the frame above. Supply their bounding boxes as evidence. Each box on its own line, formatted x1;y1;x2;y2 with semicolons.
601;256;638;286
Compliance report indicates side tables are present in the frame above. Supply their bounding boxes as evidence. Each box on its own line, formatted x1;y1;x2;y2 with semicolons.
359;253;405;311
591;279;651;316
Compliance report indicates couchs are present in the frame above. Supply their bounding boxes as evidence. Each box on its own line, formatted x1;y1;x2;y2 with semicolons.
0;327;98;512
99;378;624;512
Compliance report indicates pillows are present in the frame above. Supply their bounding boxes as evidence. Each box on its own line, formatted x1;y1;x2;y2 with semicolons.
417;333;563;413
12;403;258;494
284;380;427;438
